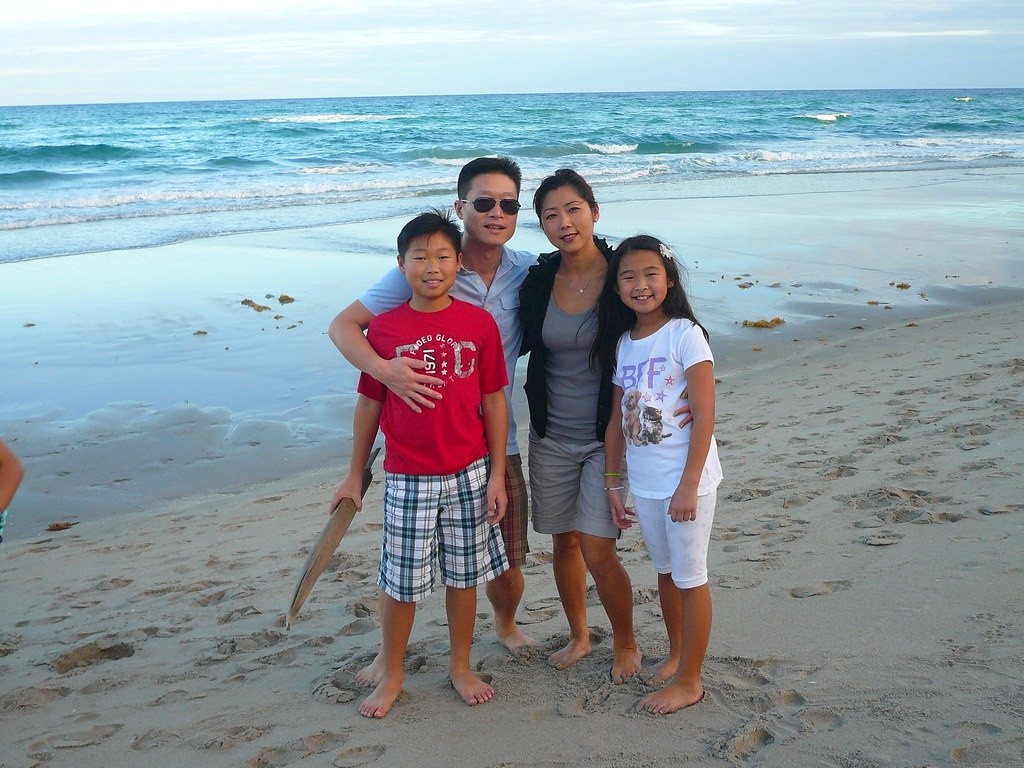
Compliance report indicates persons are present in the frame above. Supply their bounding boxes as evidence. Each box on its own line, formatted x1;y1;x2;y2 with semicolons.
590;236;725;714
330;208;507;717
0;441;25;543
330;158;541;687
518;167;693;686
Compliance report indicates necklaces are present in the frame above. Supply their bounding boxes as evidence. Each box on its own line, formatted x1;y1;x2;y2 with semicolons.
566;253;597;294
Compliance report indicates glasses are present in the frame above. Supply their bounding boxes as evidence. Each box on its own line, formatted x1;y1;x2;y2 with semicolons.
461;197;522;215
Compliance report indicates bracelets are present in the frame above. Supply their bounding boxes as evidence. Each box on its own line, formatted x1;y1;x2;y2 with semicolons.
603;473;621;477
604;486;625;491
